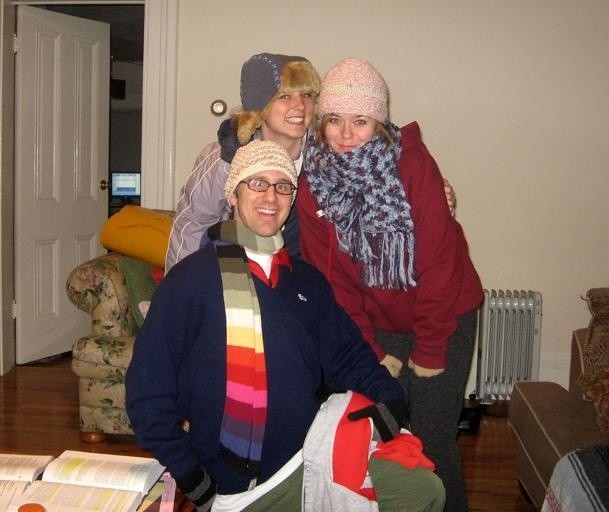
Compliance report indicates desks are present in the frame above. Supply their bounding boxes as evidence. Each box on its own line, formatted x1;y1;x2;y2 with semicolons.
136;468;194;512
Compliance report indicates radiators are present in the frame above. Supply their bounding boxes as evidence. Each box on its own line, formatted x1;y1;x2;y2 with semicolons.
476;289;542;405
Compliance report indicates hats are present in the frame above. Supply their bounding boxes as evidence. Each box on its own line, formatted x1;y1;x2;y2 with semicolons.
317;57;389;127
232;53;322;146
223;139;299;209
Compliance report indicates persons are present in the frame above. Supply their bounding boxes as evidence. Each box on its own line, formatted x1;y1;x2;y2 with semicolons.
126;138;450;512
159;51;458;284
294;57;485;512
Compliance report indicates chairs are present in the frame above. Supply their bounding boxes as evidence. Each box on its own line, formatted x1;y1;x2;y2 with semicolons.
508;328;609;512
540;442;609;512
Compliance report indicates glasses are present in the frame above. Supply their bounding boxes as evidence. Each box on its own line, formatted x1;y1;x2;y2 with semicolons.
240;178;298;195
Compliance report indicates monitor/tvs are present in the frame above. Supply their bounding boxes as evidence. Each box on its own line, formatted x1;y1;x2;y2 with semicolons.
110;171;141;197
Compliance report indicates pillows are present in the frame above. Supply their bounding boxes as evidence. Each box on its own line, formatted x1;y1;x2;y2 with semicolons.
100;204;176;329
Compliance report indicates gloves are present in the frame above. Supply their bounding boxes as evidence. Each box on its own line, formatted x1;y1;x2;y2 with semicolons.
348;399;408;441
179;466;218;511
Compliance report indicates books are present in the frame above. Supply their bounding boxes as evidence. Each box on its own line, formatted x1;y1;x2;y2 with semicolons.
0;453;54;512
6;449;167;512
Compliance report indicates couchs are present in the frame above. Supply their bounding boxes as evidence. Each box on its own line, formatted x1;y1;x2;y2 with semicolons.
66;250;141;443
